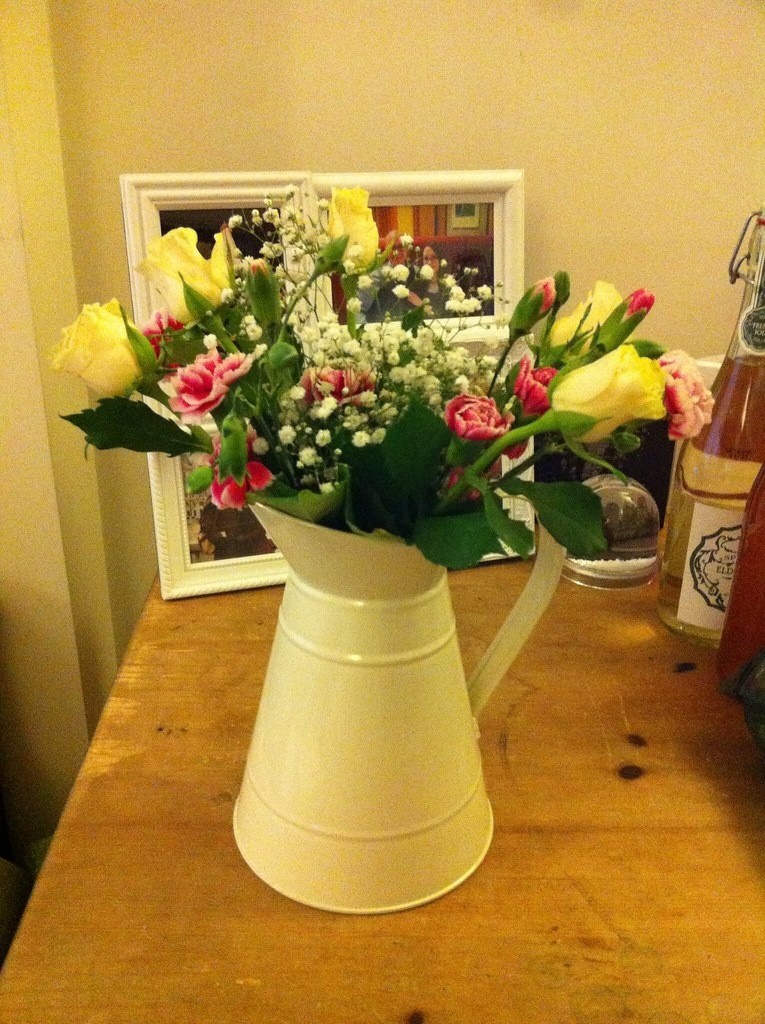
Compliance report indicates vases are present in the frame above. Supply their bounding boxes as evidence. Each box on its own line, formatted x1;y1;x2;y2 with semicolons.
231;495;495;914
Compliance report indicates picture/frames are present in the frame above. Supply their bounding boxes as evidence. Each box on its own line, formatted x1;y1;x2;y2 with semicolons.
119;166;536;600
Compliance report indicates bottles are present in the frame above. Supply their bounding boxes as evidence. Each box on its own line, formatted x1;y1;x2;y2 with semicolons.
716;461;765;681
656;208;765;646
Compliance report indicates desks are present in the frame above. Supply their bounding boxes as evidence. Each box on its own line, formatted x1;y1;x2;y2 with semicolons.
0;547;765;1024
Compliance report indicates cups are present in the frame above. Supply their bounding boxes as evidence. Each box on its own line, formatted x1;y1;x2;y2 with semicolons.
560;416;675;589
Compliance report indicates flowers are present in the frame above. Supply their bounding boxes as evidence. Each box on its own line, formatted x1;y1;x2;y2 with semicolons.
51;183;715;572
